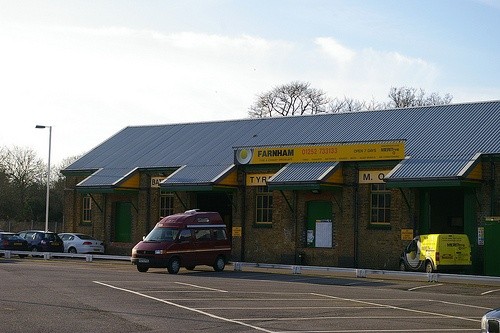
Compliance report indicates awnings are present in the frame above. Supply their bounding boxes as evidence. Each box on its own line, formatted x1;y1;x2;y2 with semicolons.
383;156;486;209
75;169;141;213
157;165;239;210
266;161;345;213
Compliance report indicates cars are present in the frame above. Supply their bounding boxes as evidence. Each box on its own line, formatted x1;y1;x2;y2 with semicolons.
57;233;105;259
0;231;30;259
18;230;63;260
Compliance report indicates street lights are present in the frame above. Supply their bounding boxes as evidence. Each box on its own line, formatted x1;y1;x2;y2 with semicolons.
35;125;51;238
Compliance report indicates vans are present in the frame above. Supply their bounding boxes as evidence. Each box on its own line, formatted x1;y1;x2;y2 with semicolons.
398;234;471;273
131;207;232;275
